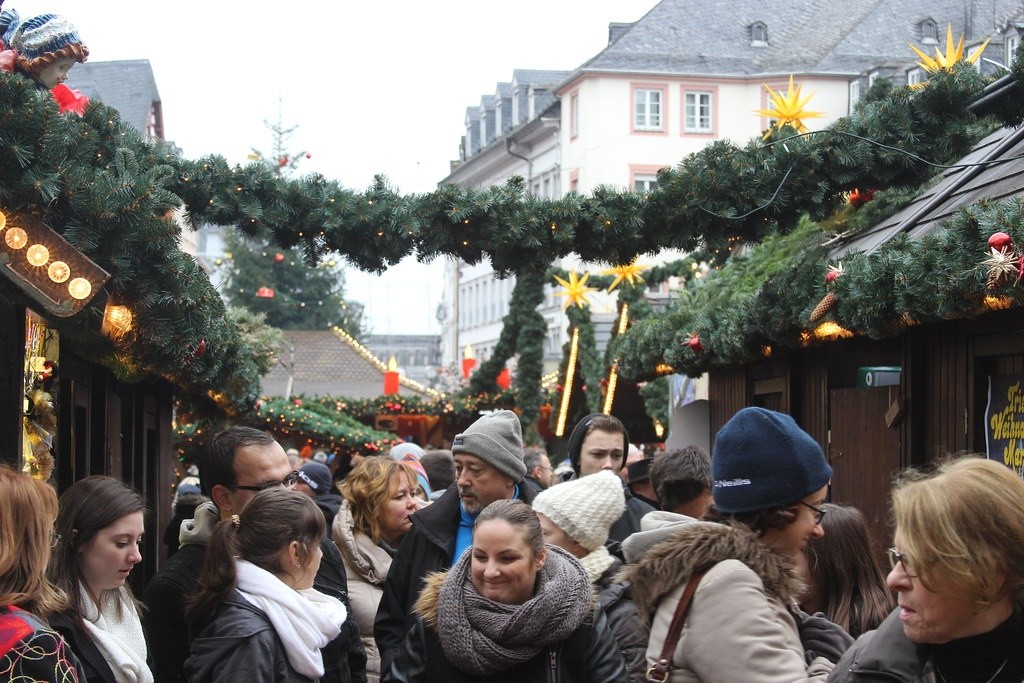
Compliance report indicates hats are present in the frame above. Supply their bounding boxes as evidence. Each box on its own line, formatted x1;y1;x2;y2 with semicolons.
178;484;201;496
298;463;333;495
390;442;426;461
287;448;299;455
710;406;832;512
314;451;327;462
567;413;629;475
300;446;312;459
397;454;431;502
452;410;527;484
531;470;626;551
627;459;654;484
327;454;336;464
187;466;199;474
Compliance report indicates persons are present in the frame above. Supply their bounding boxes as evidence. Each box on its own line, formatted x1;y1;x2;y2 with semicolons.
0;409;897;683
9;14;90;90
827;453;1024;683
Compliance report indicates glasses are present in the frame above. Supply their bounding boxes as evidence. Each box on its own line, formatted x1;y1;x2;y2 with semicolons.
231;470;299;491
800;500;827;524
539;466;553;473
888;548;953;577
50;534;61;547
